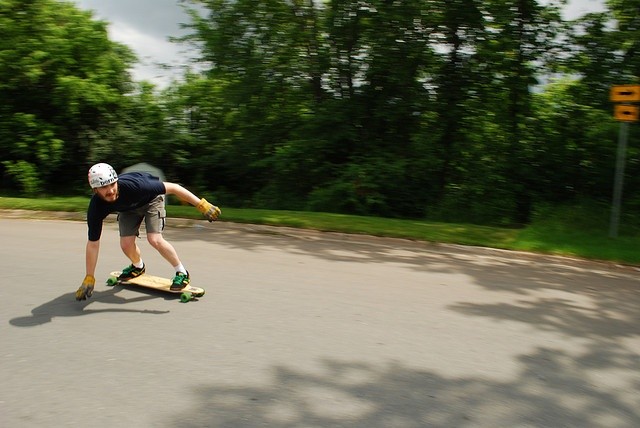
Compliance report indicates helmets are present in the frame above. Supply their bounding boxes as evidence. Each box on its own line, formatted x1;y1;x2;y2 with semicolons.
88;163;118;189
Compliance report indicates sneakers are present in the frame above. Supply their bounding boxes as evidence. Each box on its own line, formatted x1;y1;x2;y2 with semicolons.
119;263;145;280
170;269;191;290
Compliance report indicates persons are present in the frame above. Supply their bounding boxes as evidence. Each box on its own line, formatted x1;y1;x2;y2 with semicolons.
75;161;222;302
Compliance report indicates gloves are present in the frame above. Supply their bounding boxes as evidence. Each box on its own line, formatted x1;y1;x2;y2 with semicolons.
75;275;95;301
196;198;221;222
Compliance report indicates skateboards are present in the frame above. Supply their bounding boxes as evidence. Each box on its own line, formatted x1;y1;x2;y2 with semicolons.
106;271;205;302
